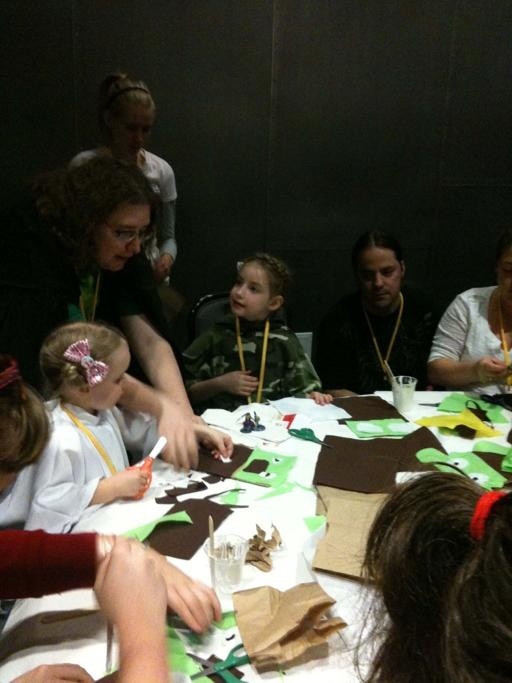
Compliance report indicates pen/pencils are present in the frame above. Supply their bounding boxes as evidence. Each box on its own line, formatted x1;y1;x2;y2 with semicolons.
381;360;411;385
207;511;217;579
106;623;113;673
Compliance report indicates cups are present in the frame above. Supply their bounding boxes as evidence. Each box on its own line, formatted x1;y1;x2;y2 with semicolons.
204;534;253;590
389;375;419;413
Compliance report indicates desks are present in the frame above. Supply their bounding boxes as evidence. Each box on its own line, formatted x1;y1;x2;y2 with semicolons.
0;389;511;683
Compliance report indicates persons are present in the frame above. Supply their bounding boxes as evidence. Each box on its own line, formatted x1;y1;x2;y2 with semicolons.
1;354;227;635
352;465;512;682
5;528;172;682
310;228;445;395
0;320;236;527
62;70;188;328
181;252;337;416
2;148;220;479
421;237;512;398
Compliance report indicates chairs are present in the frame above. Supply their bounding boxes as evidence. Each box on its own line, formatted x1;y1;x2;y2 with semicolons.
189;288;230;341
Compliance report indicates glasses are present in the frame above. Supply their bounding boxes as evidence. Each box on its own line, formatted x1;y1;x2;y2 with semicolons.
104;220;156;241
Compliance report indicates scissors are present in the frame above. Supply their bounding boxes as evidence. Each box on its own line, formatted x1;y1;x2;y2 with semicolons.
121;437;169;498
